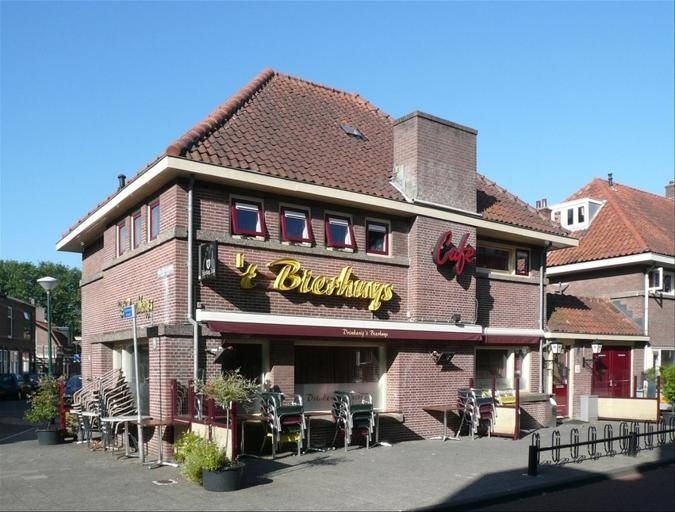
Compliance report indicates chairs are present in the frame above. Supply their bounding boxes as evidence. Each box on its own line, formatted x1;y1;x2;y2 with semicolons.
456;388;499;440
331;390;375;452
257;392;307;458
70;368;137;454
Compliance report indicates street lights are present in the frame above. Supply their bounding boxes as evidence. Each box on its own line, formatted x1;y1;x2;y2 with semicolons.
123;305;135;320
35;276;59;377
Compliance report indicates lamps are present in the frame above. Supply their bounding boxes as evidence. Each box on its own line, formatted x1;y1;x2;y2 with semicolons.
204;348;231;364
428;350;455;364
451;314;460;322
583;339;603;367
542;342;563;369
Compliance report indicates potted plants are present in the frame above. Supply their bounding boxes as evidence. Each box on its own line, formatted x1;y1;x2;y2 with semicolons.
659;366;675;425
193;378;246;492
225;367;261;414
23;373;61;444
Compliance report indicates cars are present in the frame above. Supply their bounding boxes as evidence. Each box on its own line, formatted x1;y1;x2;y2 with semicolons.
26;372;47;388
62;374;82;399
0;372;28;396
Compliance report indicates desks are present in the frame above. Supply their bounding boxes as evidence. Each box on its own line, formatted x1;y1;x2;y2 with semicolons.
303;411;332;454
235;415;272;460
132;419;176;469
422;405;466;442
373;408;402;449
